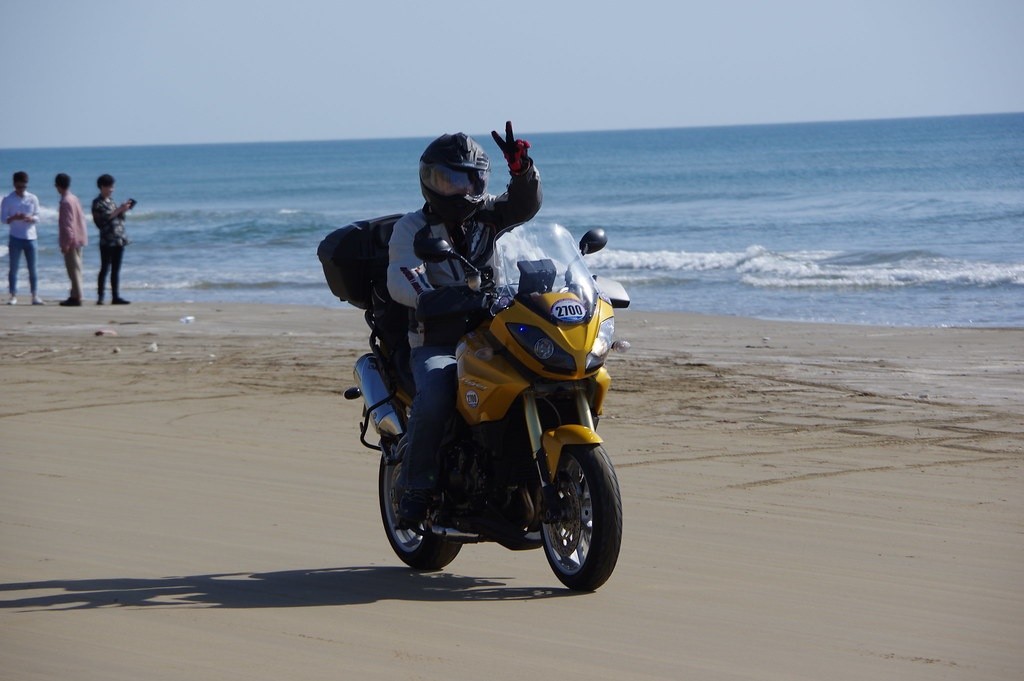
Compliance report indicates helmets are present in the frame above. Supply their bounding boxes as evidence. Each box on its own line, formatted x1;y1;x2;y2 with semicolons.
420;133;491;216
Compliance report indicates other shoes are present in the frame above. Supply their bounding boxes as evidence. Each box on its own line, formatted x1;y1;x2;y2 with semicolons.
112;297;131;305
7;295;17;306
97;296;103;304
32;296;47;306
59;298;82;307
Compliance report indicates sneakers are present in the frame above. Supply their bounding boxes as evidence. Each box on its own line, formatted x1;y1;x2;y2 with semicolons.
401;487;432;521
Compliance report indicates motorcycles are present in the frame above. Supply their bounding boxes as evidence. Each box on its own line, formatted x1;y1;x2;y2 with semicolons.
343;219;630;593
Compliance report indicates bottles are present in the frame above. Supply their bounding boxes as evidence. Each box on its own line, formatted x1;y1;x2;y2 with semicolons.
180;316;195;324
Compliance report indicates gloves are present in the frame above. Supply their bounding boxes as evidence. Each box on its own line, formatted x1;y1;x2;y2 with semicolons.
492;120;531;175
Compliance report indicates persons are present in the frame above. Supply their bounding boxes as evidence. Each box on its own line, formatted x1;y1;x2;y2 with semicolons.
54;174;88;306
0;171;44;305
386;121;542;521
92;174;132;305
